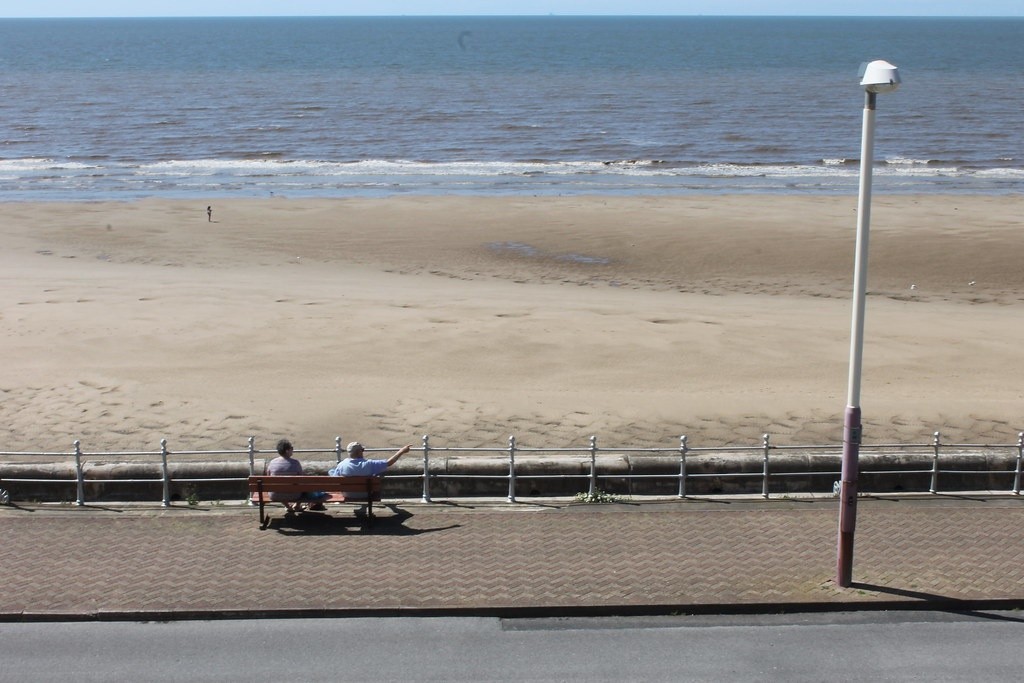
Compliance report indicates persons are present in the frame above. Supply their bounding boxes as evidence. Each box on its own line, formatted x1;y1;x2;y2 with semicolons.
207;206;212;222
264;438;305;514
326;441;414;515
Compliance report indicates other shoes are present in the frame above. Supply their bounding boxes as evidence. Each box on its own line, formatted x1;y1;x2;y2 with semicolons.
284;505;306;512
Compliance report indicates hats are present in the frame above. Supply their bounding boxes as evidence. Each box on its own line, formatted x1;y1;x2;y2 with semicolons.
346;442;366;453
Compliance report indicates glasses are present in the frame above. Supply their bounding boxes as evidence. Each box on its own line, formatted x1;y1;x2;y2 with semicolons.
289;447;294;450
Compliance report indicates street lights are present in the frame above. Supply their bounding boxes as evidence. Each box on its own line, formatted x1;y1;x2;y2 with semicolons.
835;59;903;587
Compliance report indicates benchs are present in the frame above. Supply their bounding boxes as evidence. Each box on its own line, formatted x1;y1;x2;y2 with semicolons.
247;476;383;530
